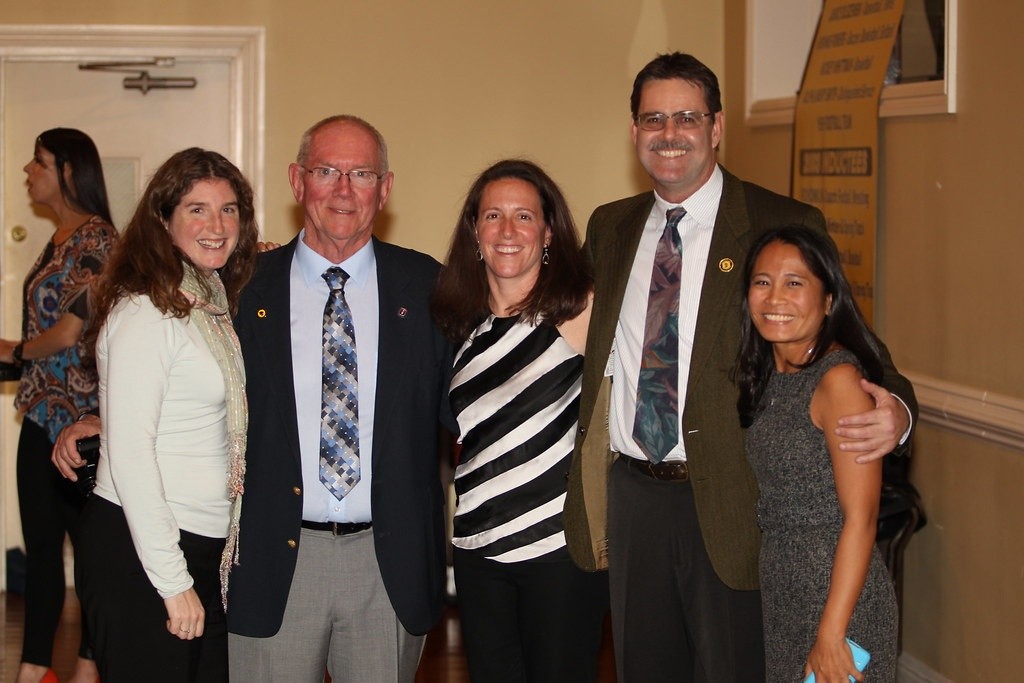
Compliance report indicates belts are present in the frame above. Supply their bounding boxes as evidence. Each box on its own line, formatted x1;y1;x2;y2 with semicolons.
300;520;371;537
618;451;690;482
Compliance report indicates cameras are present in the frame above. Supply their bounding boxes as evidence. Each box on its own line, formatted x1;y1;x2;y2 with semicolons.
72;434;101;496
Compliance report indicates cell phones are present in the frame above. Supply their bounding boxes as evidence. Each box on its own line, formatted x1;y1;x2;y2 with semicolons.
804;638;870;683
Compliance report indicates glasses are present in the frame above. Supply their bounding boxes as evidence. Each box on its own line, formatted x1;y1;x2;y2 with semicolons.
299;165;383;188
634;109;714;131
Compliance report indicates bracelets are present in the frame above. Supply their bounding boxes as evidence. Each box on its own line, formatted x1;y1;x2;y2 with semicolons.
12;343;30;364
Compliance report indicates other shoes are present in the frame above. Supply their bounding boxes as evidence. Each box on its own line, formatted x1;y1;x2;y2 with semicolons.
39;667;60;683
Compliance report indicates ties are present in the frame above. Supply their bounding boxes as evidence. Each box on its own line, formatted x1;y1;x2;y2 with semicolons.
631;207;687;466
319;266;361;499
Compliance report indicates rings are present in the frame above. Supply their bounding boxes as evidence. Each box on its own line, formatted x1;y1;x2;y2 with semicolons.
180;629;189;633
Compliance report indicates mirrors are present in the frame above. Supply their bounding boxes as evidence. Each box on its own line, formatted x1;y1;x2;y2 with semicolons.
738;0;960;130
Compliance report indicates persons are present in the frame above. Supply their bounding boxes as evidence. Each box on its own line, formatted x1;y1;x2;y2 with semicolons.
562;50;918;683
73;146;282;683
729;225;898;683
429;159;607;682
0;127;120;683
51;109;460;682
